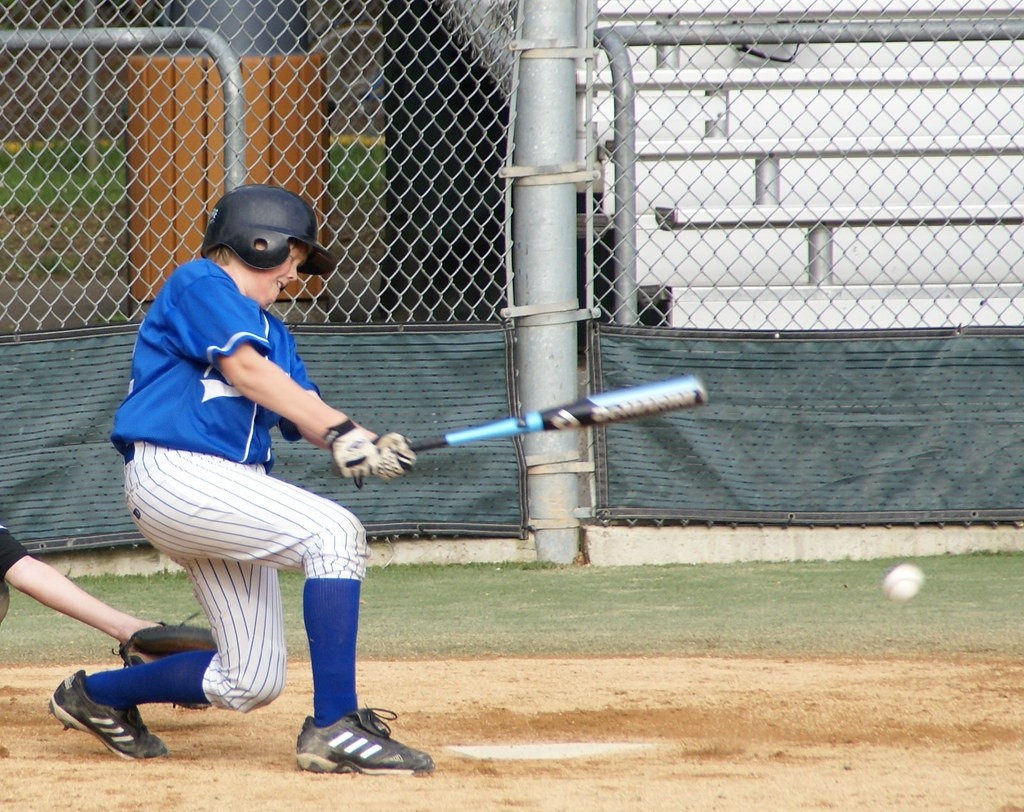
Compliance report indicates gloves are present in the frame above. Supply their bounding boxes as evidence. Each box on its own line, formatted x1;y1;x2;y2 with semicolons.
371;432;417;482
322;419;380;478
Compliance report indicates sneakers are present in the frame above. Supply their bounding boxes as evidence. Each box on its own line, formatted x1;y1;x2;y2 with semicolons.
48;670;168;761
295;708;436;776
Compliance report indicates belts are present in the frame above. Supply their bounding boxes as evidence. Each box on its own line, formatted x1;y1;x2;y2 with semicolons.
122;442;135;466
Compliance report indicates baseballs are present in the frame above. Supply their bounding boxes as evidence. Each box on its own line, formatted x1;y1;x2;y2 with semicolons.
881;563;922;601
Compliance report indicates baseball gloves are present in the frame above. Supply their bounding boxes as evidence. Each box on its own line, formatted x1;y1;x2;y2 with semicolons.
121;625;215;713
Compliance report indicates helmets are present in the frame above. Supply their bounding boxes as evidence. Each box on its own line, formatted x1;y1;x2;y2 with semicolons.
201;184;338;292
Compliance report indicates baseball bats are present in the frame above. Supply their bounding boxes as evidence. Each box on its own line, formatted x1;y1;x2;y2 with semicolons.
402;372;709;454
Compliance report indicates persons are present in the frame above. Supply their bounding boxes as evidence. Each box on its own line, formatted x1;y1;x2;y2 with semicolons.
0;522;218;710
50;184;435;779
